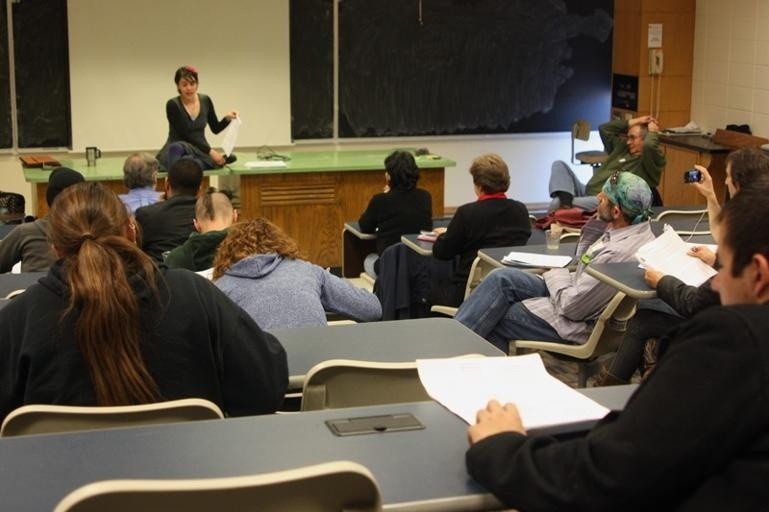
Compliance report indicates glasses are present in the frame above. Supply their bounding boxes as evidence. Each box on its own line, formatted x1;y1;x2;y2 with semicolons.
625;134;643;141
610;169;620;196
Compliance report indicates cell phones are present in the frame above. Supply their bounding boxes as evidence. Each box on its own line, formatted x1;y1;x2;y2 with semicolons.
682;169;705;186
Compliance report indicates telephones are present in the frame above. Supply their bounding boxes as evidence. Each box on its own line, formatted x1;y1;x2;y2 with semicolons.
648;47;665;77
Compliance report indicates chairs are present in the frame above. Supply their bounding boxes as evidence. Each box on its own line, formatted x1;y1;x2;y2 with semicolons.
655;210;710;237
557;233;583;247
300;353;485;414
359;272;376;294
0;397;223;438
53;460;383;512
513;290;637;388
571;119;609;172
430;256;494;318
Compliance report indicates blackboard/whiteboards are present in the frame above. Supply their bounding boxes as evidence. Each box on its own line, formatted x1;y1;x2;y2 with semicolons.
66;0;297;155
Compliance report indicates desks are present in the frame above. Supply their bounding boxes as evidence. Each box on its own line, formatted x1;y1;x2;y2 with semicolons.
654;128;735;209
477;235;717;274
0;385;639;511
263;319;508;398
401;224;565;255
342;218;451;280
584;262;662;299
23;154;230;219
223;147;458;269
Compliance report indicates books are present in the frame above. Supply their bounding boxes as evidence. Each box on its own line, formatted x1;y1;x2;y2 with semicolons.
416;227;448;243
19;156;61;167
658;119;705;137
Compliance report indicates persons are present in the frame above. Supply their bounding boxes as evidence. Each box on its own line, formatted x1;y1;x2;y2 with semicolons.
0;181;289;417
155;65;240;173
0;167;86;274
212;216;383;331
426;153;532;319
465;173;769;512
453;171;654;355
593;146;769;387
117;151;164;220
135;158;203;263
164;184;242;272
358;150;433;280
549;115;668;211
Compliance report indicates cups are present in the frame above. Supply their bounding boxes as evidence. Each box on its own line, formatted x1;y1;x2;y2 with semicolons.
162;252;170;262
86;146;101;166
546;230;559;249
